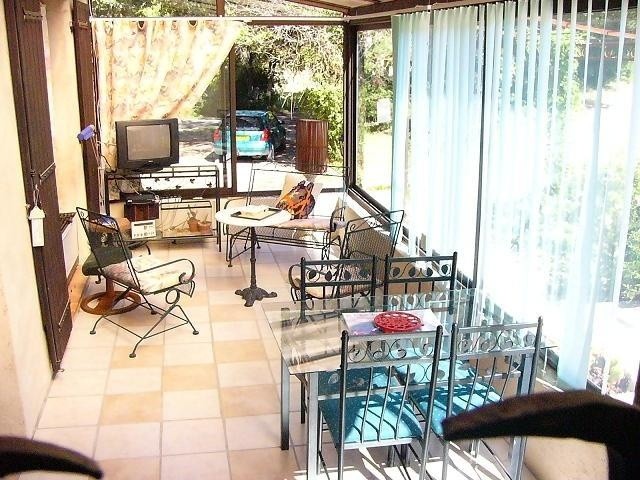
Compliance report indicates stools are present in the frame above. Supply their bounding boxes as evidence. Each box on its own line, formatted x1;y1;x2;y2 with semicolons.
80;218;141;315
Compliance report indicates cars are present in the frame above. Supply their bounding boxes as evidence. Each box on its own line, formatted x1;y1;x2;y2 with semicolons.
213;109;288;163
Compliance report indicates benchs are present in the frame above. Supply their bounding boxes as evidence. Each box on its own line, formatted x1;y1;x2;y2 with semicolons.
222;158;351;270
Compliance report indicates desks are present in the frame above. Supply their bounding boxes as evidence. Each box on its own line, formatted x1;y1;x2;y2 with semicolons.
261;287;559;480
215;205;292;307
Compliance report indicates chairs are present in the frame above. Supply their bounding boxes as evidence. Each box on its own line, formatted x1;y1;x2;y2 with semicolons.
288;211;543;479
75;207;201;358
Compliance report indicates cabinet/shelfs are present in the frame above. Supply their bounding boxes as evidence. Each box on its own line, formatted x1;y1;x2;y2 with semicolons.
103;165;222;254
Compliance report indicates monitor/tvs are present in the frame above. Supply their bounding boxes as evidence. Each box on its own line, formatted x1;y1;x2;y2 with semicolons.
115;118;180;172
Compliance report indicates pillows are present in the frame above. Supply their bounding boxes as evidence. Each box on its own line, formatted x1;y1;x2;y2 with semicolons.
272;173;326;220
